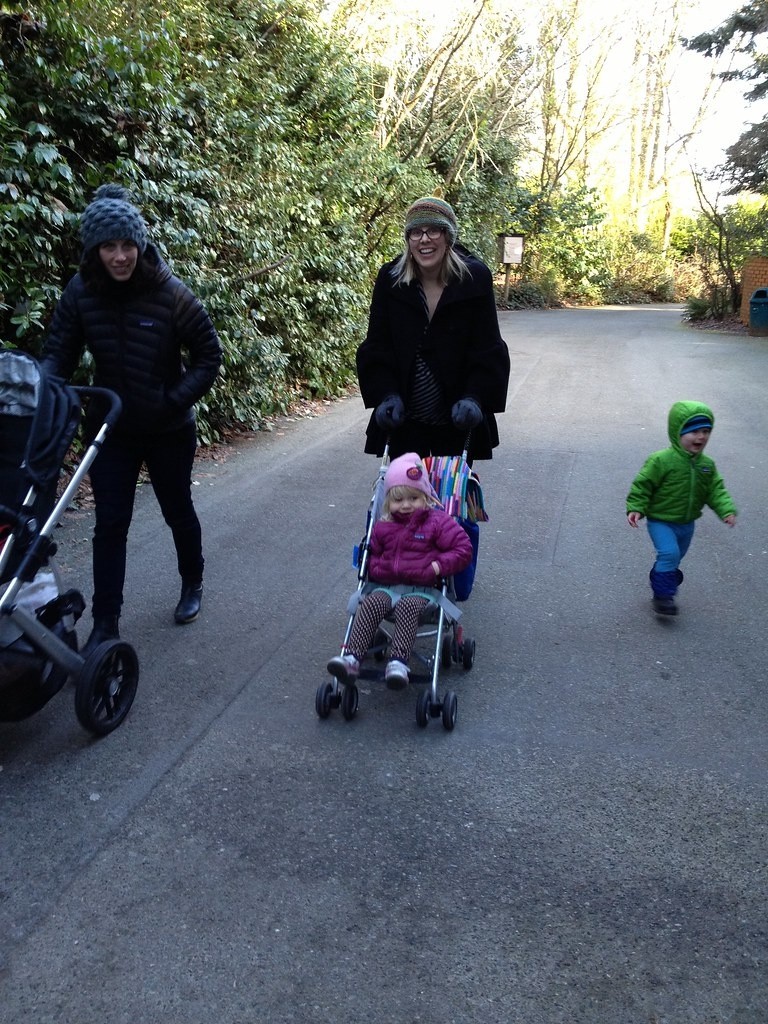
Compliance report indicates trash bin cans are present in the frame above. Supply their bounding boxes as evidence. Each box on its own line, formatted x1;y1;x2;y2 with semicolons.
749;288;768;337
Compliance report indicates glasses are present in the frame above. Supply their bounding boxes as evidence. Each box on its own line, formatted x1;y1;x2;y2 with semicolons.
406;226;444;241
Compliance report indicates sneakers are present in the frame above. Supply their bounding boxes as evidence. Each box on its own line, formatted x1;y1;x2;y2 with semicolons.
327;655;360;686
385;660;411;690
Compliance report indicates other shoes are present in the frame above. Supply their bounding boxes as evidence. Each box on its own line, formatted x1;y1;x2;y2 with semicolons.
651;595;680;617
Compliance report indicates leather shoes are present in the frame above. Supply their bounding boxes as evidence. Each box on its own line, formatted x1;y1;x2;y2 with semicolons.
175;582;203;620
78;617;122;659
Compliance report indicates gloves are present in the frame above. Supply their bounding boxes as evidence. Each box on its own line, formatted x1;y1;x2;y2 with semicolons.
376;394;405;430
452;398;483;426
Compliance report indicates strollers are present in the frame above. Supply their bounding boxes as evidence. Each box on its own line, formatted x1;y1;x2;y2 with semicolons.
316;409;479;730
0;348;138;735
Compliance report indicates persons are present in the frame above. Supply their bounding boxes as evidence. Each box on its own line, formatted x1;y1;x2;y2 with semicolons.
355;198;511;468
38;200;222;660
327;452;472;690
626;400;737;616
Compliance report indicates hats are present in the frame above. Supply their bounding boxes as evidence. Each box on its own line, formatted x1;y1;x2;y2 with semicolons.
384;452;432;496
680;416;714;436
79;182;149;254
405;187;458;249
